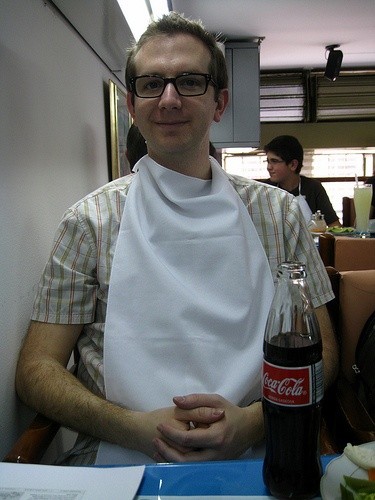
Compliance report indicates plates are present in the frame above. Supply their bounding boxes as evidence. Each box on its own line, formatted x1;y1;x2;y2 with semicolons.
320;442;375;500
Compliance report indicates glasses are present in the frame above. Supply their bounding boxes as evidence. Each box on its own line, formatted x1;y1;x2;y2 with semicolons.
128;72;218;98
263;159;283;166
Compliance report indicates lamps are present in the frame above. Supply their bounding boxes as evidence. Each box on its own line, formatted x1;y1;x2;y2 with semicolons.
323;45;343;82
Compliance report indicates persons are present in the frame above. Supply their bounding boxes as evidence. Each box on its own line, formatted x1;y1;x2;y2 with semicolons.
123;123;223;174
16;14;338;471
255;134;343;227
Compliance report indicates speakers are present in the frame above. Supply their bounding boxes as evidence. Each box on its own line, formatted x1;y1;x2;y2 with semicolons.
323;50;343;81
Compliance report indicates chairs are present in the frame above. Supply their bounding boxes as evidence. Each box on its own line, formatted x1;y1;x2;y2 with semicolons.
318;234;375;454
343;197;373;228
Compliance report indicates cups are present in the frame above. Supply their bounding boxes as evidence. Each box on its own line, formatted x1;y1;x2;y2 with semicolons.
354;183;374;237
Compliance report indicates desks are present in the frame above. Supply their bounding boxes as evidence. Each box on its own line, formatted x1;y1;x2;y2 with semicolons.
75;455;343;500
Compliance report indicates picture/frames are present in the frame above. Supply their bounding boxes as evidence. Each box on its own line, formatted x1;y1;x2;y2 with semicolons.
108;79;134;180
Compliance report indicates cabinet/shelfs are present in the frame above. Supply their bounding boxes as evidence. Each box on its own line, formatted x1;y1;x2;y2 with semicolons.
209;42;260;154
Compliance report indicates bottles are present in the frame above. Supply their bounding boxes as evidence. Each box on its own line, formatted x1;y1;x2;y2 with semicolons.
306;209;337;268
260;260;326;500
